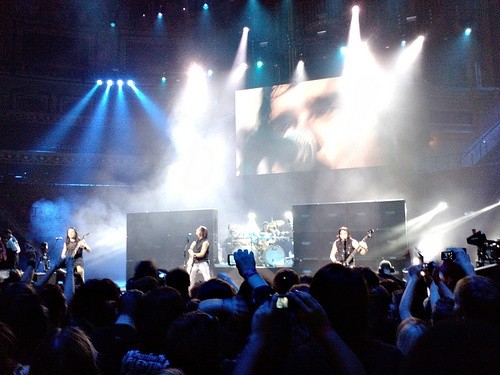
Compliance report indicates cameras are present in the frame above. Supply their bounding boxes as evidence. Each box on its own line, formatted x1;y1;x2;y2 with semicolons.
275;294;289;308
228;254;236;265
441;251;456;260
158;270;167;278
422;263;429;271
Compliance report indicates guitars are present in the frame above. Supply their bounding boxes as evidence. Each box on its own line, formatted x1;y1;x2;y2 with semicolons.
71;232;91;258
341;229;375;267
187;241;196;274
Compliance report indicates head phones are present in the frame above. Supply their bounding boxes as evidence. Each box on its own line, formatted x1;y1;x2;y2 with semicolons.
378;266;395;272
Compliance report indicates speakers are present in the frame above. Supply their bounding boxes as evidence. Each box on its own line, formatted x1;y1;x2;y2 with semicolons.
292;200;409;275
127;209;218;280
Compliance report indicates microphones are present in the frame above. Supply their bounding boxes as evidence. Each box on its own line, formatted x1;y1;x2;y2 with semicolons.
56;237;62;240
186;233;191;242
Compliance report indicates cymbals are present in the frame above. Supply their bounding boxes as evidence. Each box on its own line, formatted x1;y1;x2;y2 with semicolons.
267;220;285;228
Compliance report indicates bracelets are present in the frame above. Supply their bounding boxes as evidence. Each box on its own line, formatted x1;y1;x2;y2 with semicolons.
27;260;36;268
316;322;332;339
249;333;258;341
244;269;259;280
84;247;87;250
194;253;197;257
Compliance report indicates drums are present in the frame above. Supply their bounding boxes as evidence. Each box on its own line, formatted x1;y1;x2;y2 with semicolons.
264;244;285;266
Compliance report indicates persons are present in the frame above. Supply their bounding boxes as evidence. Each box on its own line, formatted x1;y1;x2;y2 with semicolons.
0;211;500;375
